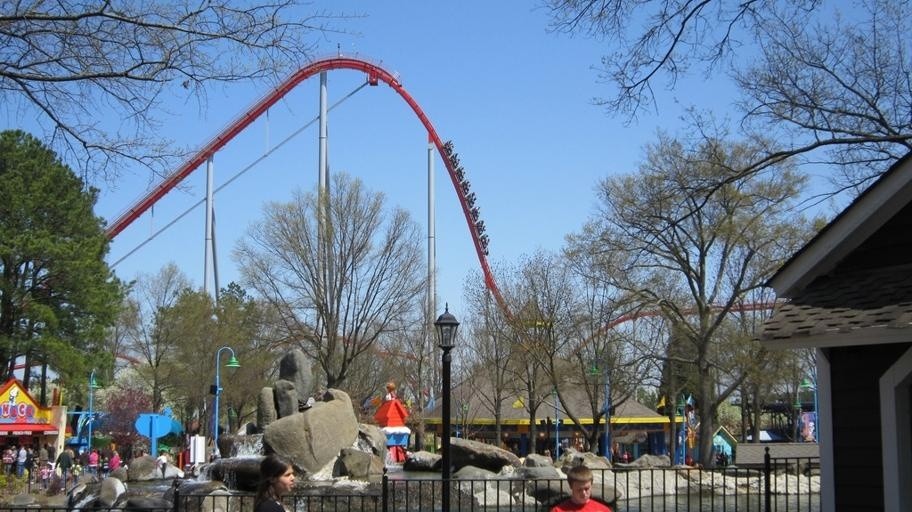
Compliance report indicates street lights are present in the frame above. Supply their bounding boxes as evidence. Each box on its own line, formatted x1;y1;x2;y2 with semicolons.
800;379;818;439
550;386;560;459
590;355;610;459
434;300;459;511
86;366;103;457
213;346;241;449
676;391;685;465
793;399;802;442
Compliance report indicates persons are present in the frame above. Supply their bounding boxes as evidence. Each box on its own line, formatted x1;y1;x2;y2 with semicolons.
251;453;298;511
0;438;174;491
547;464;615;512
